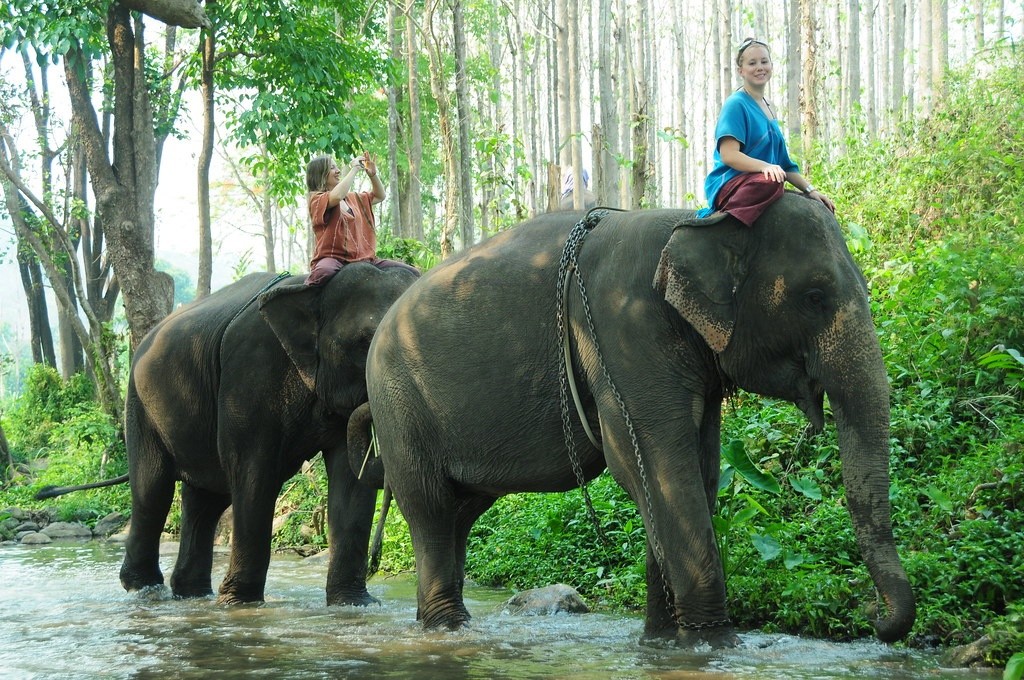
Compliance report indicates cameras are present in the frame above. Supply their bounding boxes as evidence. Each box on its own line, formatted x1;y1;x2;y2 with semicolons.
359;160;368;167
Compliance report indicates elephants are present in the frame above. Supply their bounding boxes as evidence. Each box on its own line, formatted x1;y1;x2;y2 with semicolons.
344;185;918;651
33;257;426;610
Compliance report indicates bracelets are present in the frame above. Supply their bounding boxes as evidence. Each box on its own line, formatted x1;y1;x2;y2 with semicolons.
368;173;376;178
803;185;817;196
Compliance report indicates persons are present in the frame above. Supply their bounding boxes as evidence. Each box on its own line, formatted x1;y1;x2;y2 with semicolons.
302;152;421;286
696;38;834;227
